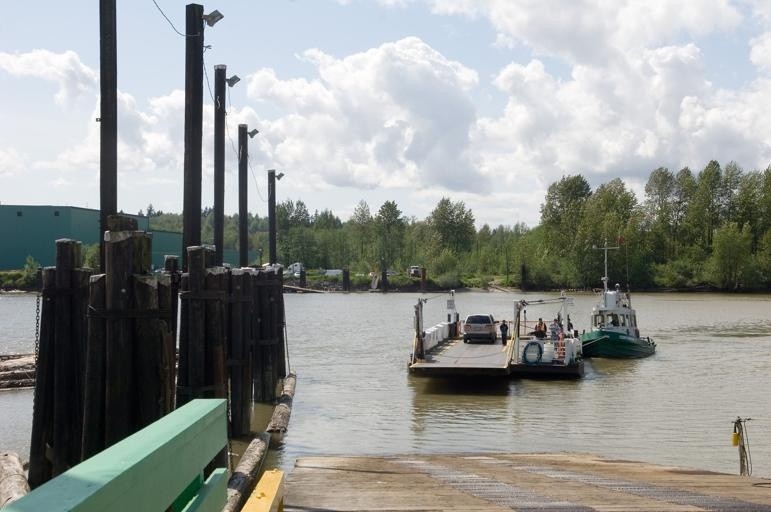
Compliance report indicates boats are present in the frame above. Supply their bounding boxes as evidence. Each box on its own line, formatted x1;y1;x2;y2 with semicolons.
582;276;656;359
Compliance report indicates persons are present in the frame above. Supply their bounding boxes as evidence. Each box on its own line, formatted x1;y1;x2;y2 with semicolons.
500;319;508;346
534;317;547;333
342;265;350;292
549;318;559;344
568;318;573;330
574;329;583;360
574;333;581;362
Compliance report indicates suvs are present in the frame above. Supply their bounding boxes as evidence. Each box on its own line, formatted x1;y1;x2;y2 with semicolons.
461;313;499;344
406;265;421;277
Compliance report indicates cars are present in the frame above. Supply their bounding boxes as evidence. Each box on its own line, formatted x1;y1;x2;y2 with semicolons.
367;271;399;277
260;262;304;278
